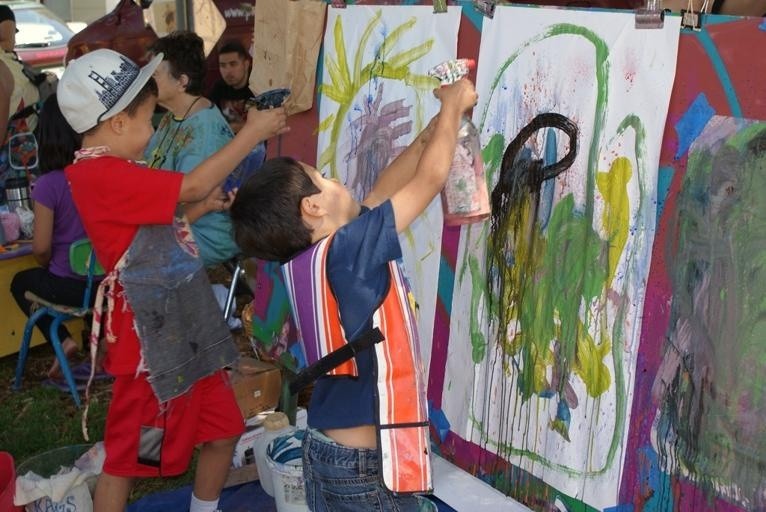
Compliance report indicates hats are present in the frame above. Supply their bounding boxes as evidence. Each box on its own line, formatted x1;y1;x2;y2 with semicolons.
56;47;166;135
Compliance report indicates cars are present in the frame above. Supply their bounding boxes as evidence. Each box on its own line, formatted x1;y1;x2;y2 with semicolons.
0;1;78;79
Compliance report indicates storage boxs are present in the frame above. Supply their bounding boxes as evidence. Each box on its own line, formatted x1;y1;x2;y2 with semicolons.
225;408;286;490
221;349;284;423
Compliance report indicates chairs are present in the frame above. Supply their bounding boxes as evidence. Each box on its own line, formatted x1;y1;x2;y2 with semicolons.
5;227;113;413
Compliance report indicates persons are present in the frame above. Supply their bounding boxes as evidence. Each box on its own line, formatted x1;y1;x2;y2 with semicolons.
228;77;478;511
2;4;290;511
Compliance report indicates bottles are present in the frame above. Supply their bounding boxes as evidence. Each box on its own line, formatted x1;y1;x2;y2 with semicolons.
254;409;301;498
4;177;33;214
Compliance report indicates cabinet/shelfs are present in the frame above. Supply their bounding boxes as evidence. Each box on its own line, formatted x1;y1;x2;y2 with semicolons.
1;239;87;366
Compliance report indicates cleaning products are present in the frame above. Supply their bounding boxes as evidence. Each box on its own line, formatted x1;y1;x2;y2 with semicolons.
424;57;495;226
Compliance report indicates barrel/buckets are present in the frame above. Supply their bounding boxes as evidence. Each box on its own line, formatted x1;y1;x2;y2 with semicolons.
253;413;296;498
264;429;311;512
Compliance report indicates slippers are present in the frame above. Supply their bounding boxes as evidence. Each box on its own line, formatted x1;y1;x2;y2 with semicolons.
39;373;87;393
71;363;107;381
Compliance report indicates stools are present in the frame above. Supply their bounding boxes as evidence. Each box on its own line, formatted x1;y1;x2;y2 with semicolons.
217;257;259;359
